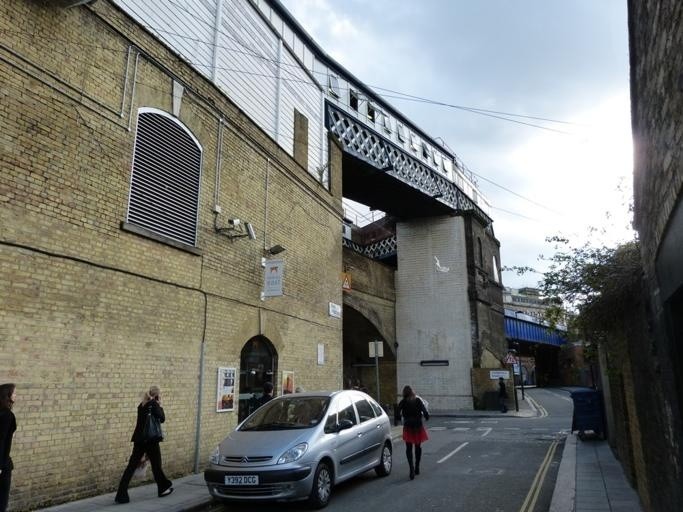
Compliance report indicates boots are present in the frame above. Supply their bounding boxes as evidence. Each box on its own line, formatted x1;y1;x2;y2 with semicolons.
405;449;414;480
413;448;421;475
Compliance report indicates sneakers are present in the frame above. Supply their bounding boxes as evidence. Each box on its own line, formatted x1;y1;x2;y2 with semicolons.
158;487;178;498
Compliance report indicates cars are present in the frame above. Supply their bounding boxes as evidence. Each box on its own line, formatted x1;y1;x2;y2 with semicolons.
203;388;394;508
238;368;272;400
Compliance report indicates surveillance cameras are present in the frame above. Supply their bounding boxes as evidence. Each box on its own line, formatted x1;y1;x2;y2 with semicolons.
245;223;256;240
228;217;240;225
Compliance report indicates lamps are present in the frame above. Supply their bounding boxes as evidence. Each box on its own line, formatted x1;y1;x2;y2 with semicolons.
261;244;286;258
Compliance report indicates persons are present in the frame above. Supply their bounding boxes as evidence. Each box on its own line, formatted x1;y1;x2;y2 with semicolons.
498;376;508;413
0;383;17;512
113;384;174;504
392;386;430;480
255;382;273;407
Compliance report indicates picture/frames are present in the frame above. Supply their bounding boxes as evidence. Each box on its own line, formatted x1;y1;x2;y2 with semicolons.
282;370;295;396
216;366;235;413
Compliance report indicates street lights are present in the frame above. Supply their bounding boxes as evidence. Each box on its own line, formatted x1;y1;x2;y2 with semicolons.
514;309;526;399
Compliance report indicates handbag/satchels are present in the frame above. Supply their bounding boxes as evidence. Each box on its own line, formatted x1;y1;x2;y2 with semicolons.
145;413;165;444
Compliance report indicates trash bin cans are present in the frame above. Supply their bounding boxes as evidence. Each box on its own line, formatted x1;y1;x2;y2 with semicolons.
569;388;602;441
